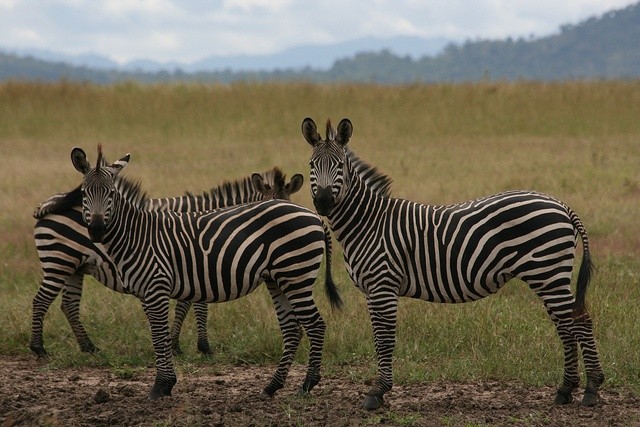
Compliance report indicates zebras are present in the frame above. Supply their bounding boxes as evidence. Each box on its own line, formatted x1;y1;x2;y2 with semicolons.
29;166;304;362
70;144;344;402
302;118;605;410
